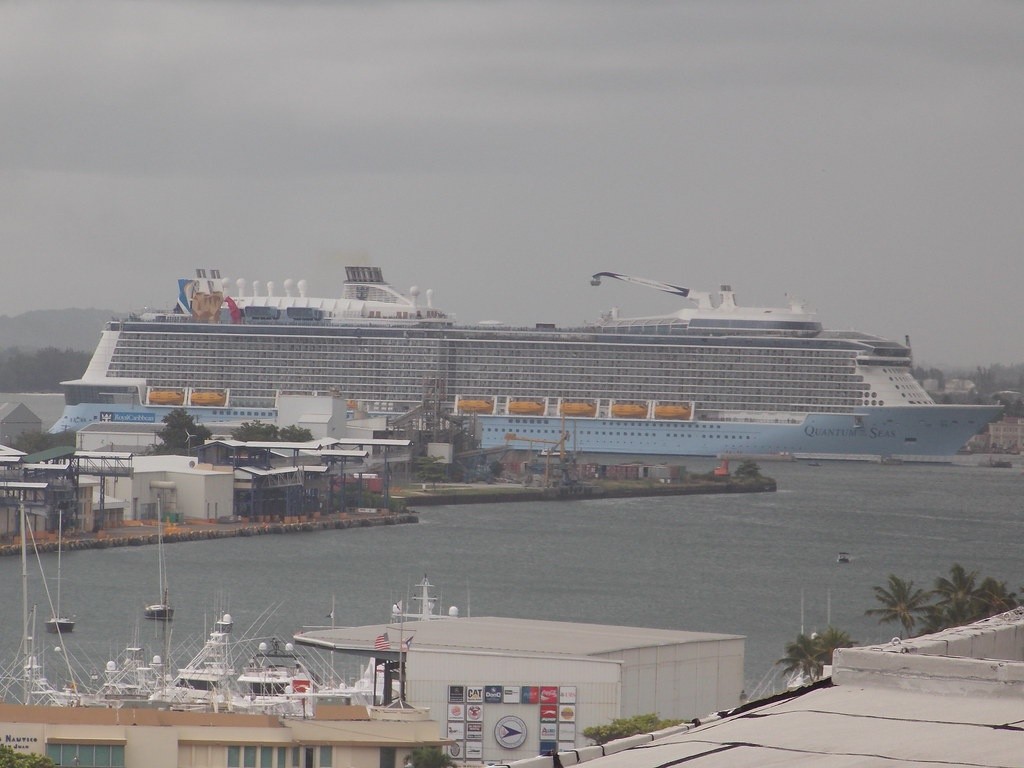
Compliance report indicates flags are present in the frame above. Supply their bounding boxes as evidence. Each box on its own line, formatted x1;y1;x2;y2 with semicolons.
372;631;392;653
404;634;416;653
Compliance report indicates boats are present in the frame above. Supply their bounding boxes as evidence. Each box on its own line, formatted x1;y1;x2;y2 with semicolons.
785;586;844;690
0;490;472;717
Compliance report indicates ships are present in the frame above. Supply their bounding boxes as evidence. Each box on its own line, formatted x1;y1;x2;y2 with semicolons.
47;268;1005;465
